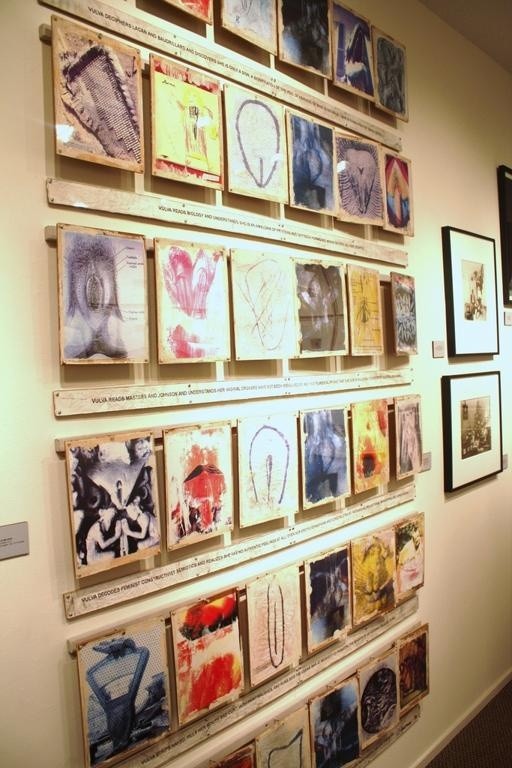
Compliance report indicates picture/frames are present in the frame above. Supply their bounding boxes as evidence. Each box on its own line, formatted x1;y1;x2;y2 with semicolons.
497;165;512;305
443;226;499;357
441;372;502;492
